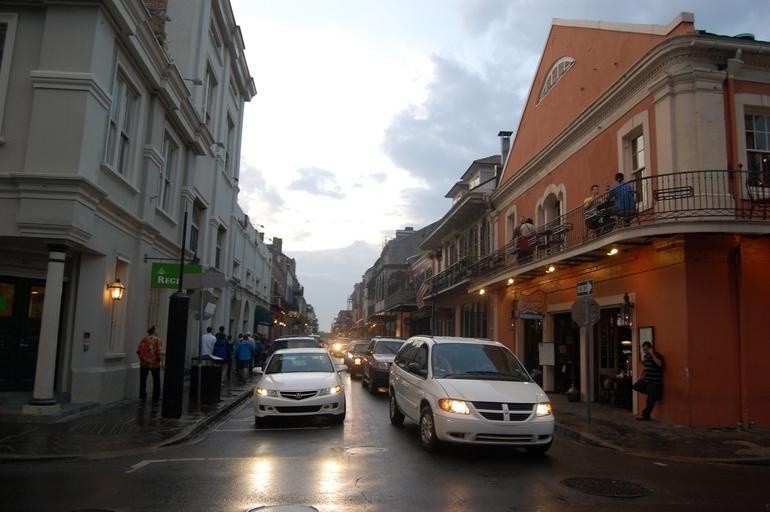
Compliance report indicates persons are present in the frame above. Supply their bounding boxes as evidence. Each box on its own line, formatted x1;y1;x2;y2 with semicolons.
630;340;666;421
602;172;636;234
583;183;616;239
518;217;536;262
511;214;526;261
200;326;270;390
135;324;165;409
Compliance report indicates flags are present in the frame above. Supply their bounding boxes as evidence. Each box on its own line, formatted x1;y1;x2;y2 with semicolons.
414;279;429;309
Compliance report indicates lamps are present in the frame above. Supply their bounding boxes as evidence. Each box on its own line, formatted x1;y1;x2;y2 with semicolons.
106;278;125;300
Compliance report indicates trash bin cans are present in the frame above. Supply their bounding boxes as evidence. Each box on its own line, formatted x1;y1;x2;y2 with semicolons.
189;354;224;404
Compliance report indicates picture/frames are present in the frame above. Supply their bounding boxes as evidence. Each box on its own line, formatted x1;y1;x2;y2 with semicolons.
538;342;556;367
638;326;655;362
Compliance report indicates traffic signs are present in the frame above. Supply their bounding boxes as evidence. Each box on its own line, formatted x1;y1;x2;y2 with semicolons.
574;279;594;298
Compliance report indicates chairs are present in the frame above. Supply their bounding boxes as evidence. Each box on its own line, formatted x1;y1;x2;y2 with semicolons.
618;190;641;226
537;230;553;258
745;178;770;220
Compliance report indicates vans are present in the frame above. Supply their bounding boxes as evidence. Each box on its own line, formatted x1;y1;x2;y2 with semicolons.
384;331;561;463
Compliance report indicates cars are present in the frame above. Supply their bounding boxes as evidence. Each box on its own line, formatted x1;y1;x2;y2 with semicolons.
267;331;405;396
252;345;353;428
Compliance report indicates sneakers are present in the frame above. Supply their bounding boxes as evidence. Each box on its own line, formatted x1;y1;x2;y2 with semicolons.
635;414;652;421
